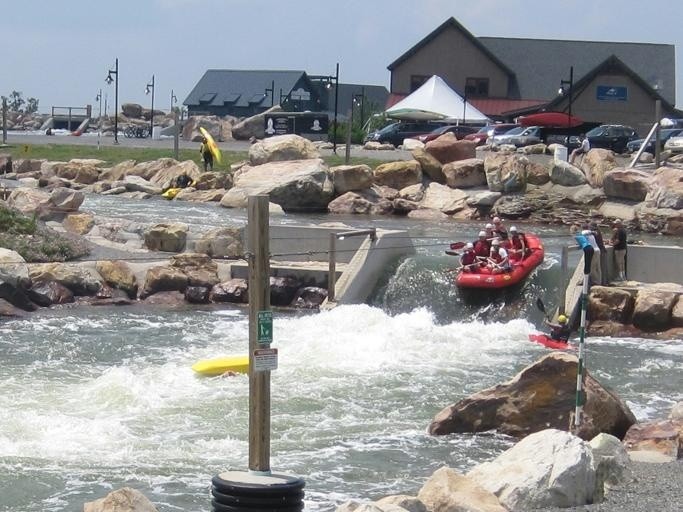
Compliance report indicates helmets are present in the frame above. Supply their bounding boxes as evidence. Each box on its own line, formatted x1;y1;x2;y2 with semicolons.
467;217;517;248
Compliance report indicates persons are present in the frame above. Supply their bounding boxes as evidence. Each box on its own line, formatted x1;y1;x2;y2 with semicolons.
46;127;51;135
458;216;513;274
570;219;627;285
543;314;571;343
570;133;590;163
509;226;530;259
199;138;213;171
173;169;193;188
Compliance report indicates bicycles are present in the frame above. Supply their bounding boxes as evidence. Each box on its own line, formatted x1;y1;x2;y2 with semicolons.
123;124;151;138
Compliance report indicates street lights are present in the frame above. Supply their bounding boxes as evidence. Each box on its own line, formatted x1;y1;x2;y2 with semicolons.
105;58;119;145
144;74;155;138
96;88;102;117
558;66;574;162
351;87;365;128
327;62;339;149
171;88;178;113
264;80;289;106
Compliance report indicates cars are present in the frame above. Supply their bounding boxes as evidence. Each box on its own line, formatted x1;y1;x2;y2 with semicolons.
417;126;478;144
626;128;683;153
465;124;540;146
585;124;639;154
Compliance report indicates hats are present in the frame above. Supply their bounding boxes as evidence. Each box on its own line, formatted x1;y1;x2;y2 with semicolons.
613;219;621;225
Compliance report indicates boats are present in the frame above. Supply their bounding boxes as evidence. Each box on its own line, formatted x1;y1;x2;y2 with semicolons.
455;232;545;289
529;333;568;350
160;181;197;199
199;126;221;166
191;356;249;377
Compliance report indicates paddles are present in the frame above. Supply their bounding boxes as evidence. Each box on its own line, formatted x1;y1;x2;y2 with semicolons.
485;256;511;272
445;251;486;259
441;261;483;274
450;237;500;249
536;298;551;323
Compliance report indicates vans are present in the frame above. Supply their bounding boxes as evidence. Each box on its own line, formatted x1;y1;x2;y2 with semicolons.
364;123;441;147
